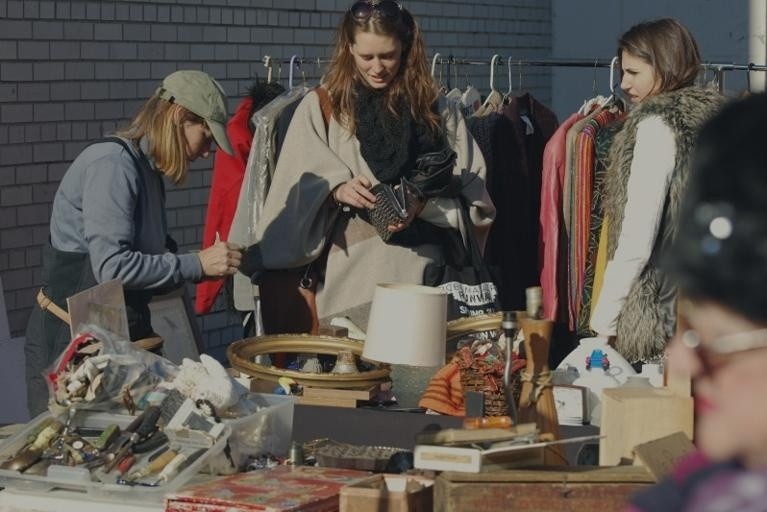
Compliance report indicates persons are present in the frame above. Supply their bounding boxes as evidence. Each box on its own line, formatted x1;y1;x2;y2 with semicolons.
584;16;724;366
624;205;765;510
24;68;243;422
254;0;497;373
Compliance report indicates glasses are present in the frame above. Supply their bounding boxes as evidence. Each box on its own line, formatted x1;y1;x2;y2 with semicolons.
673;310;766;375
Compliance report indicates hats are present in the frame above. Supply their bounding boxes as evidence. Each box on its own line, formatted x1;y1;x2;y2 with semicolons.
154;69;235;160
661;89;766;325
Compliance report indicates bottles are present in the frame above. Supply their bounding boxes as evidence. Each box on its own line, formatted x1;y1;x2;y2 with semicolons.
513;317;568;467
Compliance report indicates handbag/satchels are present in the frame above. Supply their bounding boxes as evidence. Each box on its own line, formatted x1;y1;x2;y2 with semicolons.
35;286;164;357
257;264;319;369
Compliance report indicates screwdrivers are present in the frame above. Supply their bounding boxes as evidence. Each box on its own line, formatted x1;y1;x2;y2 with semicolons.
105;406;161;474
129;447;208;485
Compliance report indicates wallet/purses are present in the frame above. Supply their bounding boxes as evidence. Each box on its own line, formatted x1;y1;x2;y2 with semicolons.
364;175;410;245
